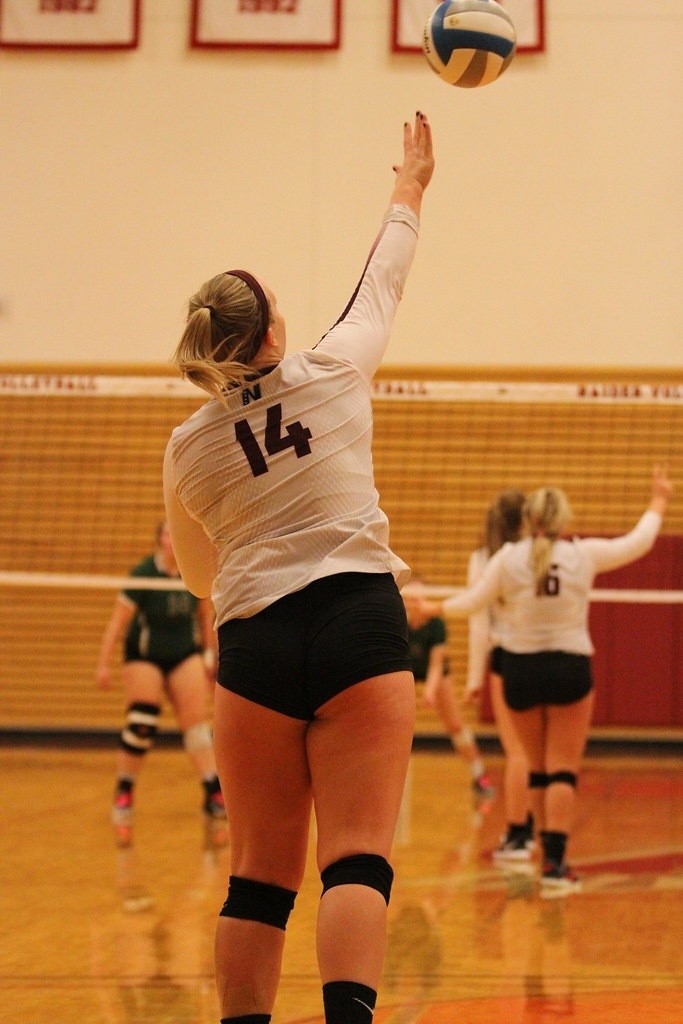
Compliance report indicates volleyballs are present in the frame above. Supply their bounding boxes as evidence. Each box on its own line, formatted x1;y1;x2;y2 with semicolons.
423;0;517;89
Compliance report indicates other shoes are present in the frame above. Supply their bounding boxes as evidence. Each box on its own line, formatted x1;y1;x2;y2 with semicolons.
112;793;131;815
491;834;533;859
201;778;227;817
539;857;582;886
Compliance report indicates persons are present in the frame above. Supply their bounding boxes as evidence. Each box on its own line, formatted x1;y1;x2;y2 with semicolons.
98;523;231;908
167;109;432;1024
399;464;673;891
